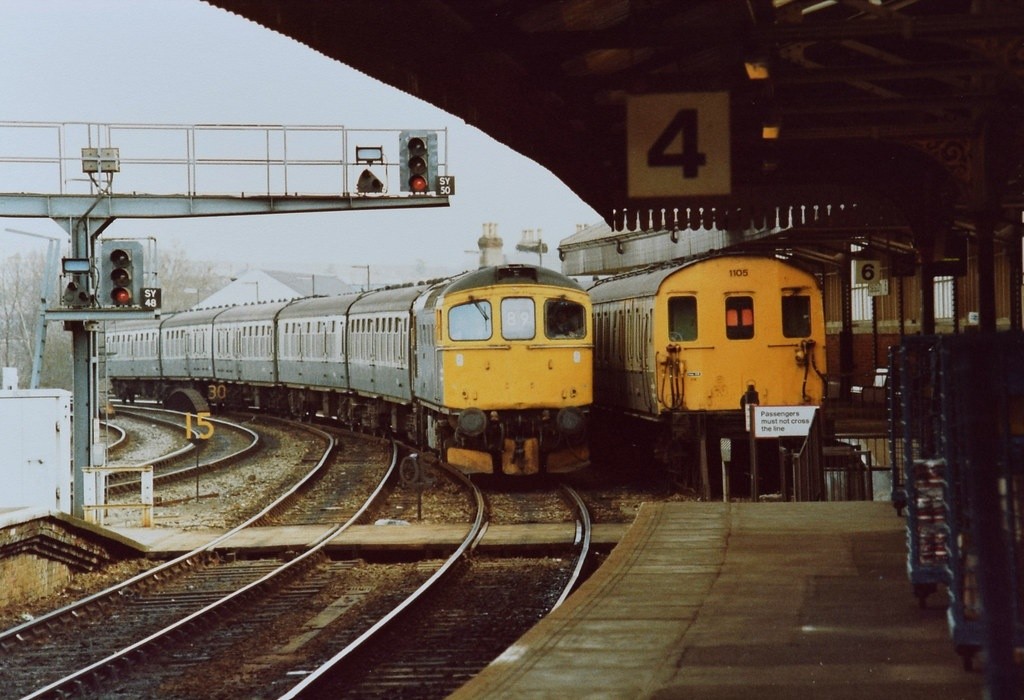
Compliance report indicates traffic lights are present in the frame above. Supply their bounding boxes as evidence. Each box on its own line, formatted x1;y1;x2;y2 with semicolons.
397;128;438;194
100;239;144;309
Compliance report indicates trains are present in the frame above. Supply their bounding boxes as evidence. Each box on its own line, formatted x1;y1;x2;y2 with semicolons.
568;250;827;492
103;264;595;480
